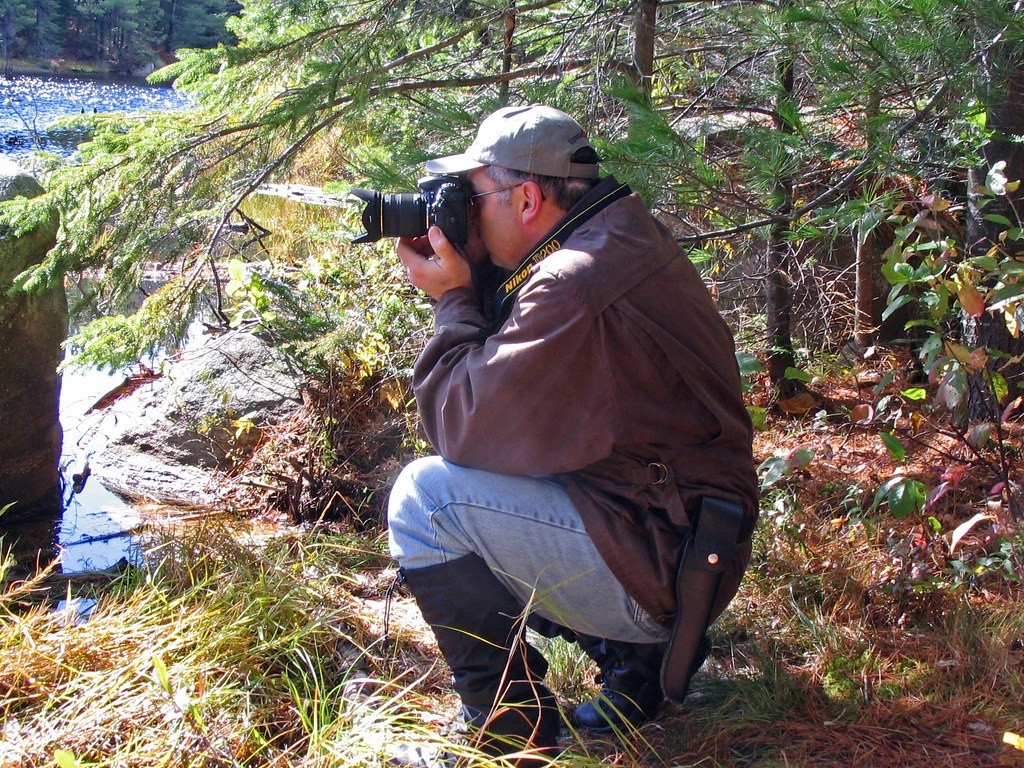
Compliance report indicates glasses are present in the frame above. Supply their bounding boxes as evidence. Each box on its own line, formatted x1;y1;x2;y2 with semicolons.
466;184;546;205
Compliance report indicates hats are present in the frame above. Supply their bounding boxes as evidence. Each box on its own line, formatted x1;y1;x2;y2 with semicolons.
426;102;600;178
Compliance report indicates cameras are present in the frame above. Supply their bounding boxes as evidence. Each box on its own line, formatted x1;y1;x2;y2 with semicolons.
351;176;469;245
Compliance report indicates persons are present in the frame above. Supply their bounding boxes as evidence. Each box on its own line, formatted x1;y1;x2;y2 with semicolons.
382;102;759;768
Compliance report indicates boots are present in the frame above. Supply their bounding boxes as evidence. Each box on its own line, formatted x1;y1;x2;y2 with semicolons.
525;612;712;733
384;552;561;768
